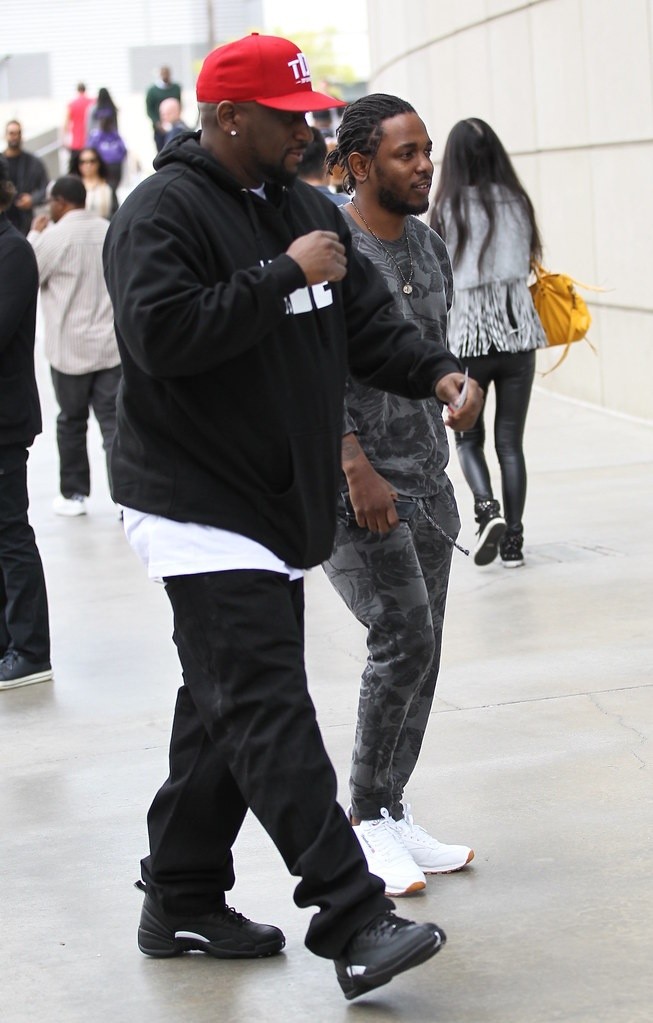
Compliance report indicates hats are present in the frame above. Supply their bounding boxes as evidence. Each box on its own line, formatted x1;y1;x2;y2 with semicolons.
196;33;348;112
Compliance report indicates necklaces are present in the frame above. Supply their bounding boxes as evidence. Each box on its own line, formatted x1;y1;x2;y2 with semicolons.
348;197;415;295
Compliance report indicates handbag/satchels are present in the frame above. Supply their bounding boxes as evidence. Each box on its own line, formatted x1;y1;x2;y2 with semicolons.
528;258;590;347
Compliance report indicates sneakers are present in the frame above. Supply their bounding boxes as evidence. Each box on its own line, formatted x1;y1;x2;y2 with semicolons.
390;803;475;875
347;805;426;896
0;651;53;690
472;500;507;566
498;536;524;567
134;880;286;958
334;912;446;1000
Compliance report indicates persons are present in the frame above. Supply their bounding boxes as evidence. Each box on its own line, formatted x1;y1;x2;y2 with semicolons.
26;176;128;522
101;33;484;1001
92;88;117;135
92;119;127;211
159;96;192;147
1;153;55;689
147;64;179;151
305;92;484;896
0;120;48;237
70;148;119;221
430;117;545;567
59;84;97;173
297;104;356;208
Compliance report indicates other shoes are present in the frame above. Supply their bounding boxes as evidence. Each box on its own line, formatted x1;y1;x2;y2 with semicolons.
53;495;88;516
